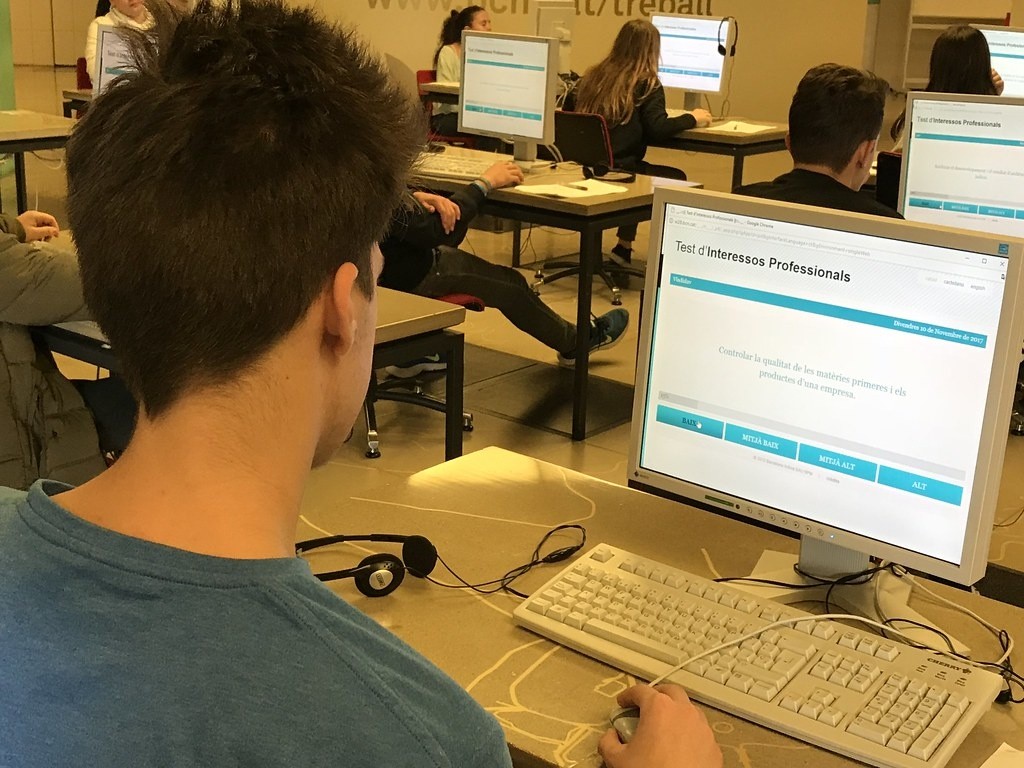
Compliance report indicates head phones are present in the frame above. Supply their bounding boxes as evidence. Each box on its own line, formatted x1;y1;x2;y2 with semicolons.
295;534;438;599
717;16;739;56
582;163;636;183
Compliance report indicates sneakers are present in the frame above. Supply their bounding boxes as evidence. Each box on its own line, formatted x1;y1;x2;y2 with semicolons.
557;307;629;364
386;352;445;379
610;244;634;268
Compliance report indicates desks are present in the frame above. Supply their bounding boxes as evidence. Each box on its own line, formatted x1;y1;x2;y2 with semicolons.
1;79;1024;768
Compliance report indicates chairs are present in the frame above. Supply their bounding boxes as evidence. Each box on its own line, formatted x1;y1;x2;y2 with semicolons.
533;108;649;307
415;68;505;153
361;294;485;459
75;58;93;121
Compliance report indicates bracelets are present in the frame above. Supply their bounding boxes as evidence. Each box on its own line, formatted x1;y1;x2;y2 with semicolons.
475;177;492;193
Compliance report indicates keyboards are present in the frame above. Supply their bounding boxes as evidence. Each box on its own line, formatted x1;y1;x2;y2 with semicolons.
513;542;1003;768
409;152;498;181
665;109;692;118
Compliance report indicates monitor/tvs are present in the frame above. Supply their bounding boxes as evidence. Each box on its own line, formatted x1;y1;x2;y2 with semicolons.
91;23;159;103
624;183;1023;659
896;91;1023;240
456;30;559;163
649;12;735;112
968;24;1024;99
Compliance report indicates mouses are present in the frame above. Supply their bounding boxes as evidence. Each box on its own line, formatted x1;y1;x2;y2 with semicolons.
609;704;640;744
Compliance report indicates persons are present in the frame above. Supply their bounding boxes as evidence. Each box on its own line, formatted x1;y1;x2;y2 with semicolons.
0;210;133;494
0;0;724;768
732;62;904;219
376;161;631;378
562;20;711;268
86;0;155;85
892;25;1004;154
432;5;492;137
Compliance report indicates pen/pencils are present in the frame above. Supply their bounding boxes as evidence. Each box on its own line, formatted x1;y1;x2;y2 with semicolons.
560;183;588;191
